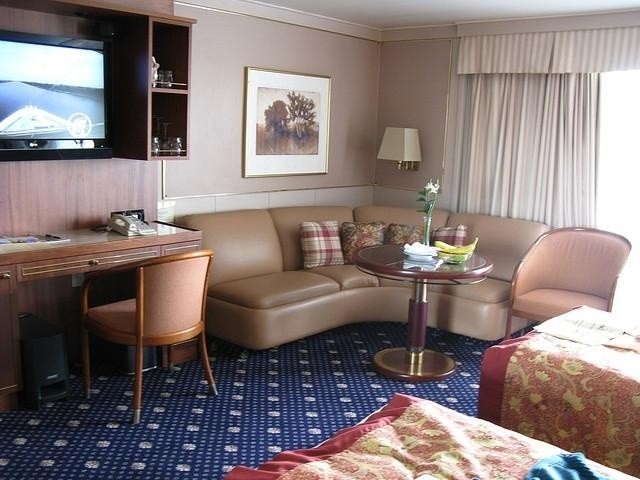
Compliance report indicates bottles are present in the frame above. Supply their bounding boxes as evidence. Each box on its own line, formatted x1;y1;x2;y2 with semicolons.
158;70;176;88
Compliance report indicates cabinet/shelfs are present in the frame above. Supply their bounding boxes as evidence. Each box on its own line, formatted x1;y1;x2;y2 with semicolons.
115;13;193;162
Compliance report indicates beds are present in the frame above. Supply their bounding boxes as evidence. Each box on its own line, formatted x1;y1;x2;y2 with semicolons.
217;395;640;480
472;300;640;477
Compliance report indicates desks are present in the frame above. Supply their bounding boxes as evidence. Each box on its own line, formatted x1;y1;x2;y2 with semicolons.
0;222;205;411
352;244;494;384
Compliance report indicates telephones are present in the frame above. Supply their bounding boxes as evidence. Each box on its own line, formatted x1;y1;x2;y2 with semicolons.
107;214;157;237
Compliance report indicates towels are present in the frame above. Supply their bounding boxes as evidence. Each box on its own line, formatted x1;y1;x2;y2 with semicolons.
403;240;444;274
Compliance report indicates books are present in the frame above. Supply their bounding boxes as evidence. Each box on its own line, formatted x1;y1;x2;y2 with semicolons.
0;230;72;247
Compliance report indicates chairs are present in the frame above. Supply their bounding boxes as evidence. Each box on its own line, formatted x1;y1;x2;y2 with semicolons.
506;226;633;343
75;249;220;425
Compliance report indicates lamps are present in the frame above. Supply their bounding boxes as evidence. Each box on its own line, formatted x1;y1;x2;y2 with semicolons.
375;123;424;176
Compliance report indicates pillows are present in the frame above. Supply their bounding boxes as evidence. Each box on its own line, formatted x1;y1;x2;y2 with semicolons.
300;215;345;270
340;219;386;269
386;221;431;245
434;224;471;246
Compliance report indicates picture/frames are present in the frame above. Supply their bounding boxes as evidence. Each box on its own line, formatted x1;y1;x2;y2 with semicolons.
241;64;332;182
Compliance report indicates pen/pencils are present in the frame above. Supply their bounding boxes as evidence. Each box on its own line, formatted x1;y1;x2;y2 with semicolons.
46;234;61;239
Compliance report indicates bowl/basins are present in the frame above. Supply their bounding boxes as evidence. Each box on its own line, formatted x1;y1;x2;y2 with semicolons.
438;250;476;265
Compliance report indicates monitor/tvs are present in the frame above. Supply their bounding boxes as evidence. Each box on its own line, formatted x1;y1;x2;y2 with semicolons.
1;29;115;160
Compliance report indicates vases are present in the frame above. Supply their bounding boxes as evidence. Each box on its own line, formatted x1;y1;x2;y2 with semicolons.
421;215;433;246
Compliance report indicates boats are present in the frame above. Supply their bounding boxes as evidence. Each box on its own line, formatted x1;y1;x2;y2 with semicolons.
0;102;94;139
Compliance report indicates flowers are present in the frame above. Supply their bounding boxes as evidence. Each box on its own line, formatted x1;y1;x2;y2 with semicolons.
414;178;444;243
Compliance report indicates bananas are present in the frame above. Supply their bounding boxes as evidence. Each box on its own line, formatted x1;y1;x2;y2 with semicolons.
434;236;479;254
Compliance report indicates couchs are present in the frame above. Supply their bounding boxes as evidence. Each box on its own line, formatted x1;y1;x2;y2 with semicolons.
175;205;552;355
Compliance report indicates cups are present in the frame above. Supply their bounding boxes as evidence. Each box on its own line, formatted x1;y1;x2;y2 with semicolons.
152;116;172;157
168;136;183;154
